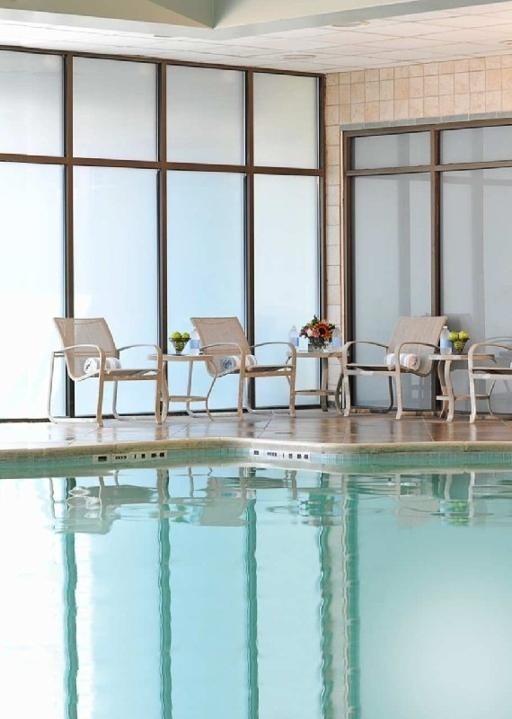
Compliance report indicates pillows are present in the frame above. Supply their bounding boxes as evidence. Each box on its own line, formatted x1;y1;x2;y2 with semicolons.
384;353;420;371
83;356;122;374
222;354;257;370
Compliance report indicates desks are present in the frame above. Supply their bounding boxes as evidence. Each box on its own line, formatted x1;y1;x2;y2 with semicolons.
428;354;498;423
146;352;219;420
286;348;347;416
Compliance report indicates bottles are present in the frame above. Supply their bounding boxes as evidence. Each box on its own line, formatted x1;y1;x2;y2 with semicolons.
190;326;200;356
288;325;300;355
439;325;453;355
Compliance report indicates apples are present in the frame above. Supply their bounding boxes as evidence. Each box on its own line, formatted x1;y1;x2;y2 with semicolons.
448;330;469;339
170;332;190;339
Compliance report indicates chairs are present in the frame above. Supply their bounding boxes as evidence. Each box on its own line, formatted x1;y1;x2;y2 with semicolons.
47;315;163;427
341;315;447;420
466;336;512;424
193;317;298;419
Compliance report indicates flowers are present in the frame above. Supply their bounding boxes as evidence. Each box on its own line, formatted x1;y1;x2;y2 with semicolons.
300;315;336;352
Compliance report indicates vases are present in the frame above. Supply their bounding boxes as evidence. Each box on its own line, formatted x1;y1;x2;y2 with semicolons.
307;343;332;353
